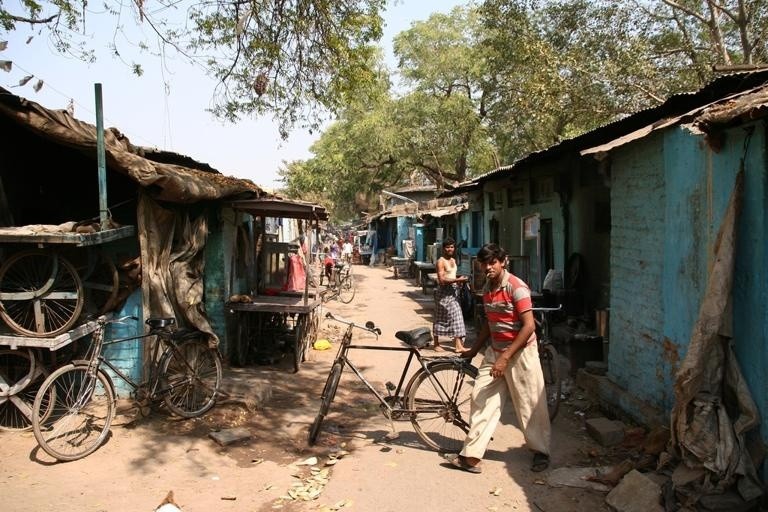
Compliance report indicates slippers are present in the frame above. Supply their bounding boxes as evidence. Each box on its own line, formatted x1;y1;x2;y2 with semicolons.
531;452;549;472
444;453;482;473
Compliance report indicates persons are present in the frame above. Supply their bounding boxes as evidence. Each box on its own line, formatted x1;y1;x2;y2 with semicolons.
433;237;472;353
300;236;308;255
445;242;552;474
323;229;354;280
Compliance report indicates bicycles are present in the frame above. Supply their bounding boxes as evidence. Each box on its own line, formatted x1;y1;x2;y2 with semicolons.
334;262;356;304
31;310;224;462
531;303;564;423
306;311;495;453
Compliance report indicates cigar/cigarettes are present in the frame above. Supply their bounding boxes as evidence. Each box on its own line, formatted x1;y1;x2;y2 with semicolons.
486;273;490;279
